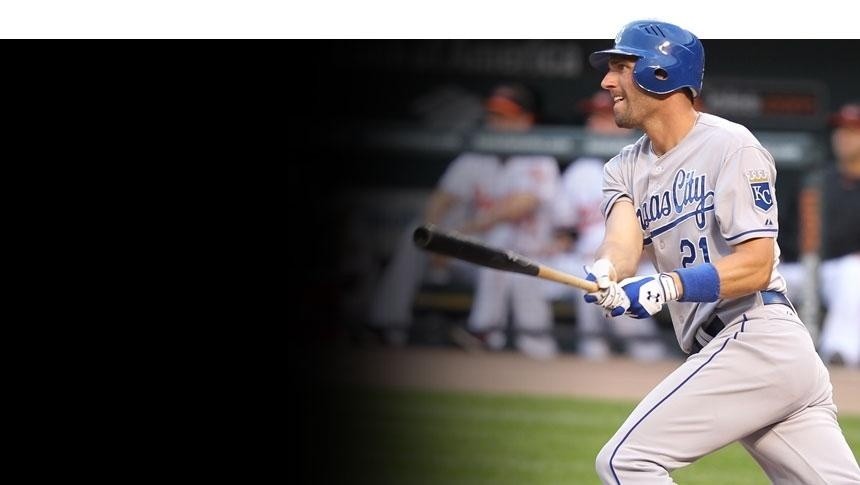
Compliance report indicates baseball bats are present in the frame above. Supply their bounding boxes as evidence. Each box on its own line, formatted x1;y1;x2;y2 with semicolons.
413;226;600;294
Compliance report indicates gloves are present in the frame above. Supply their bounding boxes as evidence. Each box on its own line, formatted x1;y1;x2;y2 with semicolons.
582;258;632;320
615;270;679;320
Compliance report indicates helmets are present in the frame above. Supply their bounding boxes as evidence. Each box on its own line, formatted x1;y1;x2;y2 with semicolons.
588;18;707;99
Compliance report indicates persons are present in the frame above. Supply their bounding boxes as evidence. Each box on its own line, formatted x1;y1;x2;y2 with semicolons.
426;89;561;363
548;79;667;364
793;102;859;364
575;19;860;483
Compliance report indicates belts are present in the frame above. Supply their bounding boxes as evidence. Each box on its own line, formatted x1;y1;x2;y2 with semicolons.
759;289;797;315
